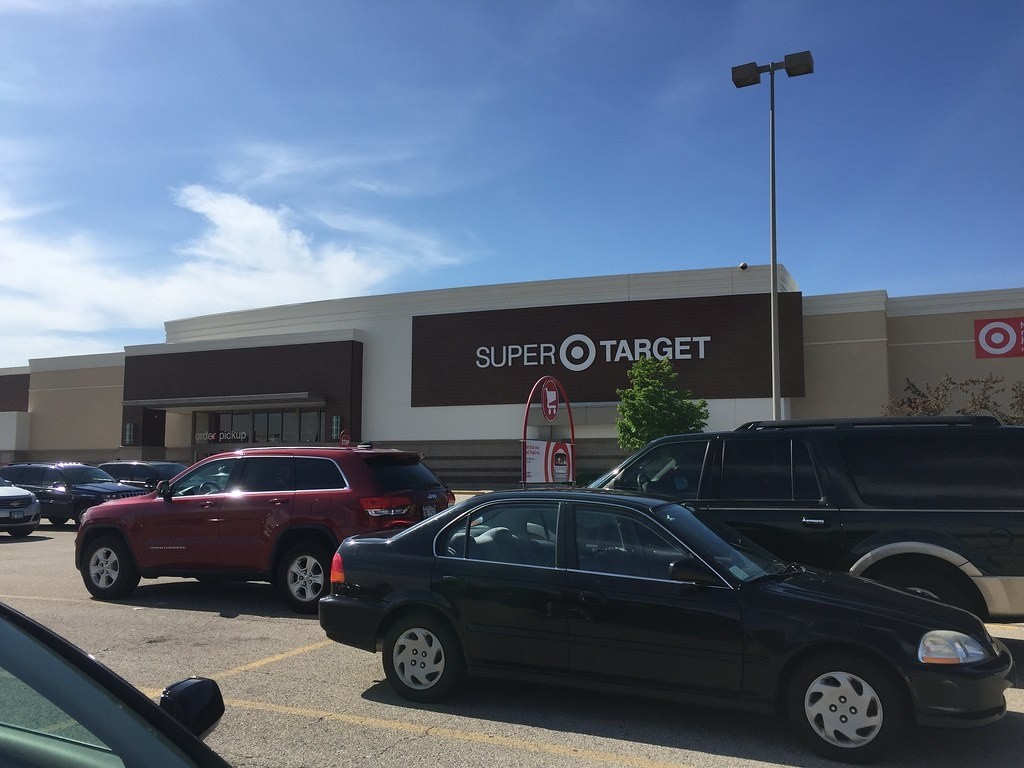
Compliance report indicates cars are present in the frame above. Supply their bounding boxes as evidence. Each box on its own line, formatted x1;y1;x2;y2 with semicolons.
0;604;230;767
317;487;1015;766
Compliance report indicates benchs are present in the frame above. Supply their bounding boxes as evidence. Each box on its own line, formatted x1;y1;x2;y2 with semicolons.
448;526;548;567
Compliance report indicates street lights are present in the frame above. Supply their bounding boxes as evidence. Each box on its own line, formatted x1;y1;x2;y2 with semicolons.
731;50;816;428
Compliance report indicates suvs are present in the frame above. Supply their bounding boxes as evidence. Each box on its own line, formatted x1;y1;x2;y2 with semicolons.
74;443;457;614
472;415;1024;625
1;461;152;527
96;459;205;493
0;478;42;540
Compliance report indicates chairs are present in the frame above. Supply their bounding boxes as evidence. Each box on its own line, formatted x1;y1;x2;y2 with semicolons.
576;523;599;571
591;521;649;577
